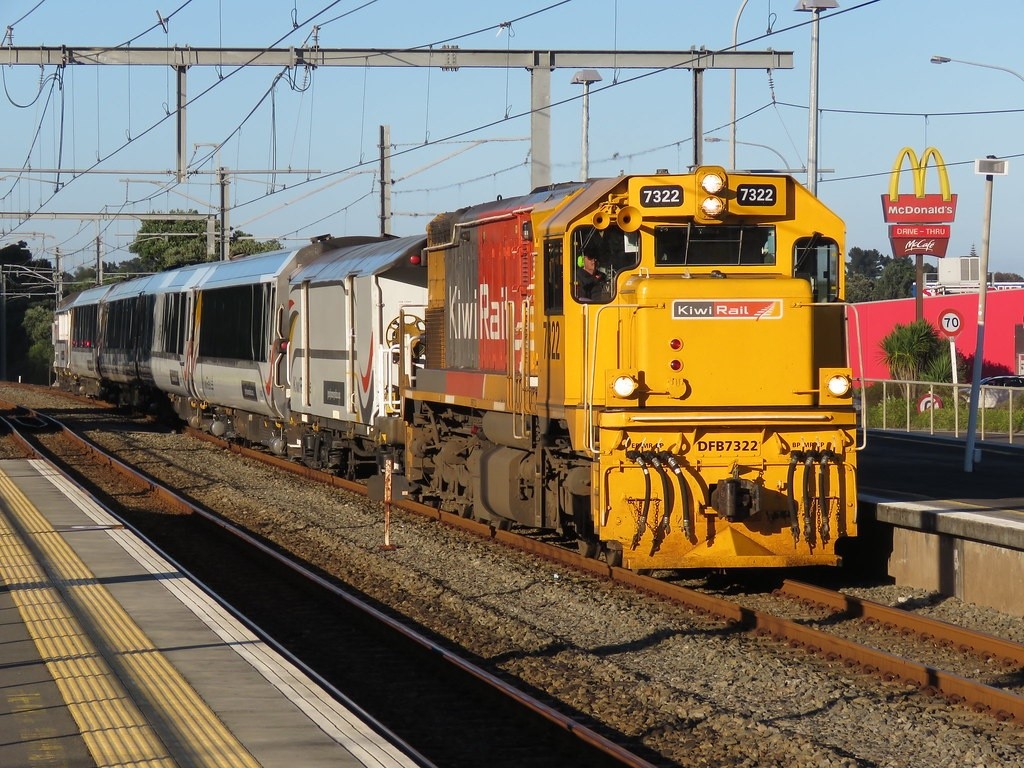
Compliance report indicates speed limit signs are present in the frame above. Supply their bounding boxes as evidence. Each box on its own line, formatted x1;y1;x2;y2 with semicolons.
938;308;965;338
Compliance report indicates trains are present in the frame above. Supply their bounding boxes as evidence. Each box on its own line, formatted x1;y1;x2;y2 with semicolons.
51;167;867;576
